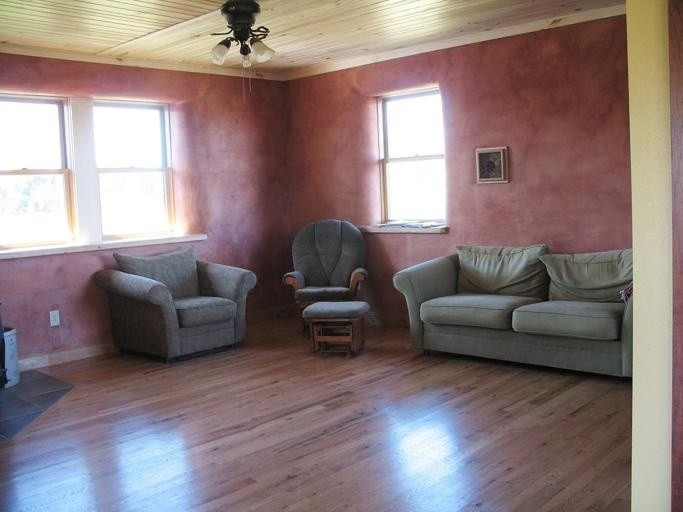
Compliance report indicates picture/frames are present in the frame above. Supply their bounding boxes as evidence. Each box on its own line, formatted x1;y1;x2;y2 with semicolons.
475;146;509;185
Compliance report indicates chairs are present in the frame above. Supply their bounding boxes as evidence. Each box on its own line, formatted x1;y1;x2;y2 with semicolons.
92;261;258;364
281;219;368;339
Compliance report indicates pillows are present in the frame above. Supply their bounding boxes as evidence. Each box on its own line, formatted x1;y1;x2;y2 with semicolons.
113;245;200;298
538;248;633;303
455;244;549;299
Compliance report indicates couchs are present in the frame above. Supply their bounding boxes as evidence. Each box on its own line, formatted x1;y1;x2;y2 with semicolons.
392;253;632;377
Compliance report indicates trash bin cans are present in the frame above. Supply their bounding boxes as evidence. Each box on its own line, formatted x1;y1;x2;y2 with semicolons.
3;327;21;389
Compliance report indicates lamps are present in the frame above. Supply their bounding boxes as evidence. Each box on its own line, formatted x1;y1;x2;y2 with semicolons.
207;0;276;103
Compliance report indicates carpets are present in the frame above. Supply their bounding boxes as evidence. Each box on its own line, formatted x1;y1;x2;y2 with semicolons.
0;368;74;444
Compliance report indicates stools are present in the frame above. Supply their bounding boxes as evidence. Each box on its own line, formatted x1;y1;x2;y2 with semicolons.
302;301;370;354
